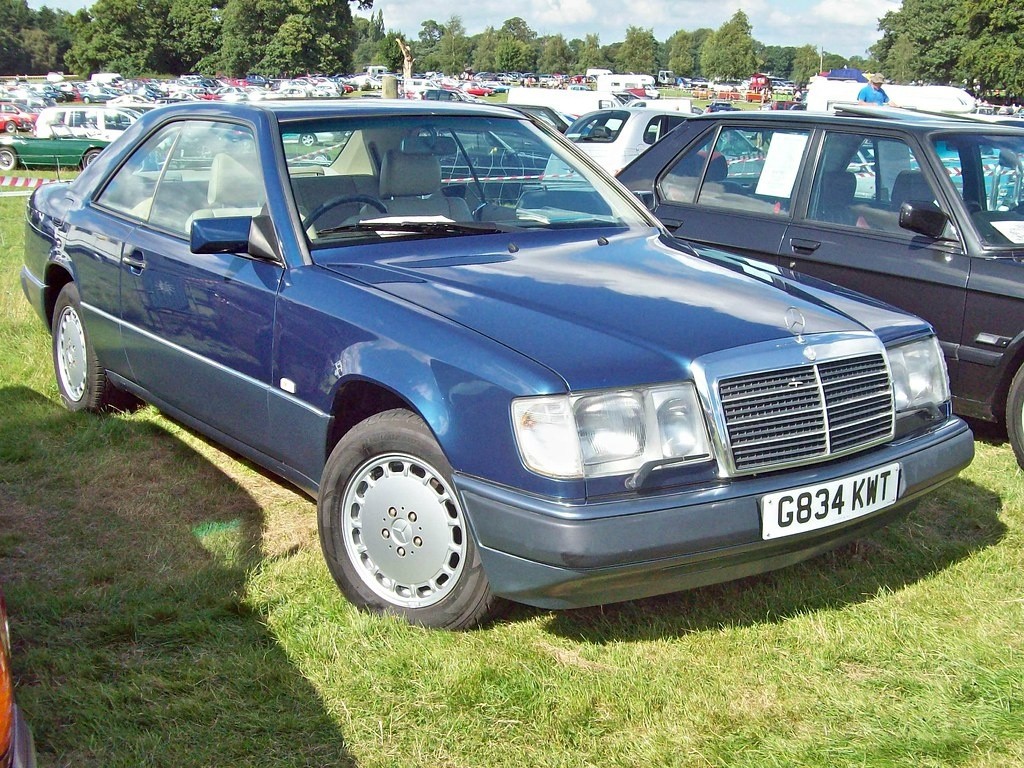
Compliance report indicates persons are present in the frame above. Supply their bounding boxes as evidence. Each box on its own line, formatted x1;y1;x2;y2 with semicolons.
760;82;772;104
793;85;802;101
856;76;897;106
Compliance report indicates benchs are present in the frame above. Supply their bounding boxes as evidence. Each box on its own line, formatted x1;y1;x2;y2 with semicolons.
684;152;791;214
129;173;379;243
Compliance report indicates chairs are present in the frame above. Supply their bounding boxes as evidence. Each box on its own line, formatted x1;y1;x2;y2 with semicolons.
357;150;474;225
890;170;936;212
185;153;317;260
814;170;860;227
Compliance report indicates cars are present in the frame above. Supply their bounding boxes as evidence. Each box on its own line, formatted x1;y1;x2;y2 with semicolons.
565;106;699;178
615;107;1024;474
21;100;976;633
1;61;811;174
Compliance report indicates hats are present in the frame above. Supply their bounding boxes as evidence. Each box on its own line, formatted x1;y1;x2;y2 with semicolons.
871;73;887;84
